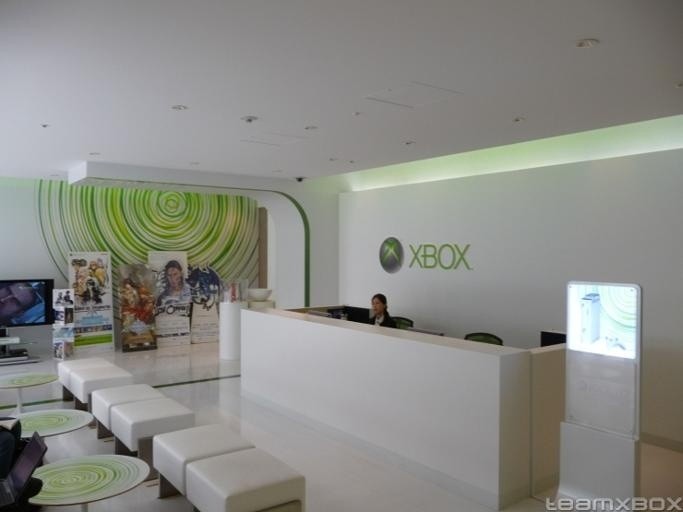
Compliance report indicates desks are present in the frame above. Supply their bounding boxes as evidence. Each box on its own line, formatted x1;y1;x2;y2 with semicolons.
1;369;150;511
0;326;43;366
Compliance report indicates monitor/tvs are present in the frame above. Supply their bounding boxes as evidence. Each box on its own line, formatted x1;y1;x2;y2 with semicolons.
0;279;53;327
344;306;369;324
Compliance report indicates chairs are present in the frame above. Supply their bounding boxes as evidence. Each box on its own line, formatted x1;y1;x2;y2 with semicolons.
464;330;504;347
392;313;415;329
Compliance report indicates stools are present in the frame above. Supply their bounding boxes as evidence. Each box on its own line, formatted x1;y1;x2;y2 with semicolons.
56;355;307;512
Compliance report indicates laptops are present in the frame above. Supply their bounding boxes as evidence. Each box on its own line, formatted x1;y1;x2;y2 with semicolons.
0;431;47;510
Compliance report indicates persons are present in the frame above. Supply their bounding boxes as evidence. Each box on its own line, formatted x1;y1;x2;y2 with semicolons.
367;294;396;328
1;282;36;321
52;258;190;360
